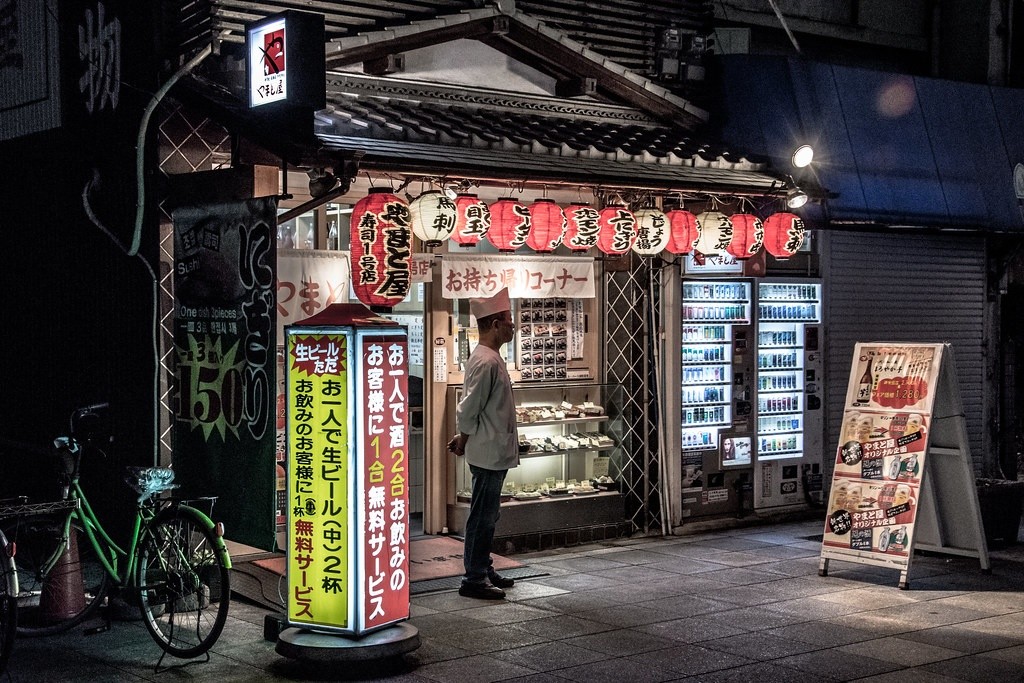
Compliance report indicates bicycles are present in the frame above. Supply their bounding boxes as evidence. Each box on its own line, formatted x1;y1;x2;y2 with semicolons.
2;402;233;675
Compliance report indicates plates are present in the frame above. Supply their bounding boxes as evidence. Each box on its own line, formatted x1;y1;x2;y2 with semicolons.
513;495;542;501
574;488;600;495
546;493;573;497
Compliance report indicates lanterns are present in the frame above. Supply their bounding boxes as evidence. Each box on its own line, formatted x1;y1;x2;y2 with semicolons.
349;186;412;313
410;189;808;260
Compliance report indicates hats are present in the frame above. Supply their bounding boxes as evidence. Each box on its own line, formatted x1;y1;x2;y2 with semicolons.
469;287;511;319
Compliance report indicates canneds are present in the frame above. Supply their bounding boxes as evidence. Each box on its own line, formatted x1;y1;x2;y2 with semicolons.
682;284;746;444
758;284;817;451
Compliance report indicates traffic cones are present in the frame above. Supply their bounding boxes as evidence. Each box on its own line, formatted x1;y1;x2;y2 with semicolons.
16;485;101;633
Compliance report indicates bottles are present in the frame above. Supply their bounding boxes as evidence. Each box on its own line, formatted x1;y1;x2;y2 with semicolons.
889;455;902;480
857;357;873;402
682;283;747;447
878;526;890;551
895;526;906;544
906;454;918;471
758;284;817;451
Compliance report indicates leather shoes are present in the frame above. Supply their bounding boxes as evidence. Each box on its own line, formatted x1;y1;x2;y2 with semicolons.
459;577;506;599
487;572;514;588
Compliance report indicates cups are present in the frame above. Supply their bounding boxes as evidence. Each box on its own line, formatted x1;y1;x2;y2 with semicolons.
546;477;555;488
847;493;862;513
891;487;910;507
568;479;577;486
556;480;566;490
505;482;514;493
844;423;858;442
857;417;873;443
904;414;923;436
582;481;589;492
831;490;848;513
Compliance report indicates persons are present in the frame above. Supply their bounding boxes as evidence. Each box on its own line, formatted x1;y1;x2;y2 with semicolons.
446;285;522;601
